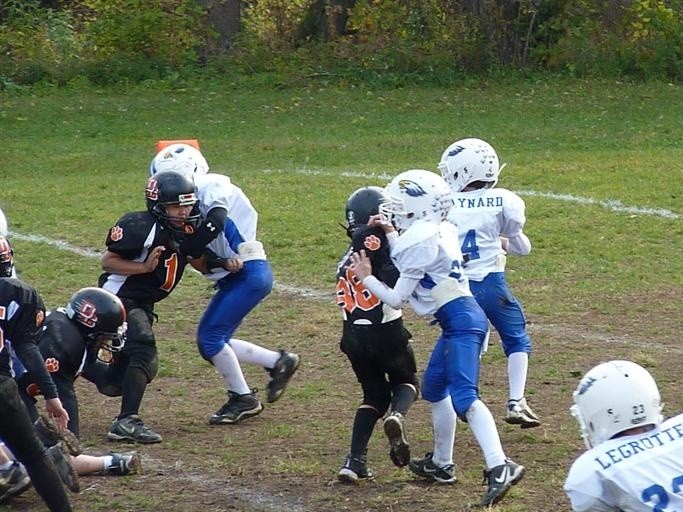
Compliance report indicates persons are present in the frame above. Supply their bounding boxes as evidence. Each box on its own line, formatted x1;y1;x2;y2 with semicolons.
98;171;243;446
437;139;540;429
0;212;142;512
335;185;420;481
563;359;683;512
148;144;300;426
343;170;526;506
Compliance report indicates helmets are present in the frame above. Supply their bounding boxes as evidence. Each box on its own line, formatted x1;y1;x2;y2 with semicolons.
65;287;128;364
346;169;453;232
439;136;507;193
145;143;210;234
1;207;15;278
569;359;667;449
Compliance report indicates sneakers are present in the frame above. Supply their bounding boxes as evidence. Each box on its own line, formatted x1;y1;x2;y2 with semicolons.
264;349;302;402
384;410;411;467
108;415;162;443
409;451;458;484
106;449;141;474
478;457;527;506
338;453;383;488
504;396;540;429
209;387;265;423
0;460;31;502
34;413;83;493
